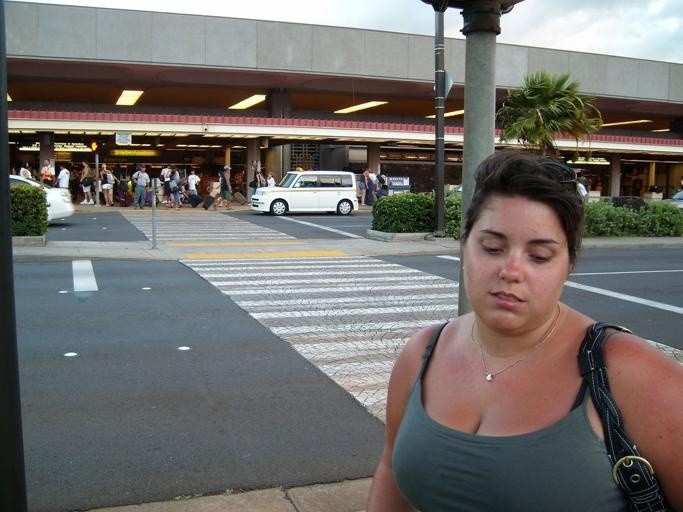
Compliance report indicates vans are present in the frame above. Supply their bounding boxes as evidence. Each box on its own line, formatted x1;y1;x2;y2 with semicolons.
249;168;359;216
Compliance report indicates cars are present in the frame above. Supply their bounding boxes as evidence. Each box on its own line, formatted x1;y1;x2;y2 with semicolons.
664;188;682;211
9;173;74;226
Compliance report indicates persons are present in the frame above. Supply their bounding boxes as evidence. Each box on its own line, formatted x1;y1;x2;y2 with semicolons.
12;159;276;212
357;167;388;206
672;185;683;200
367;149;683;511
305;179;313;187
576;179;588;202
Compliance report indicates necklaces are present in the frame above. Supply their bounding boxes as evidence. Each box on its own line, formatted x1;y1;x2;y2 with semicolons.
470;302;561;382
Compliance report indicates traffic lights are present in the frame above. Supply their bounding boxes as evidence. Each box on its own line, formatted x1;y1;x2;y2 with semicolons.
89;138;106;154
